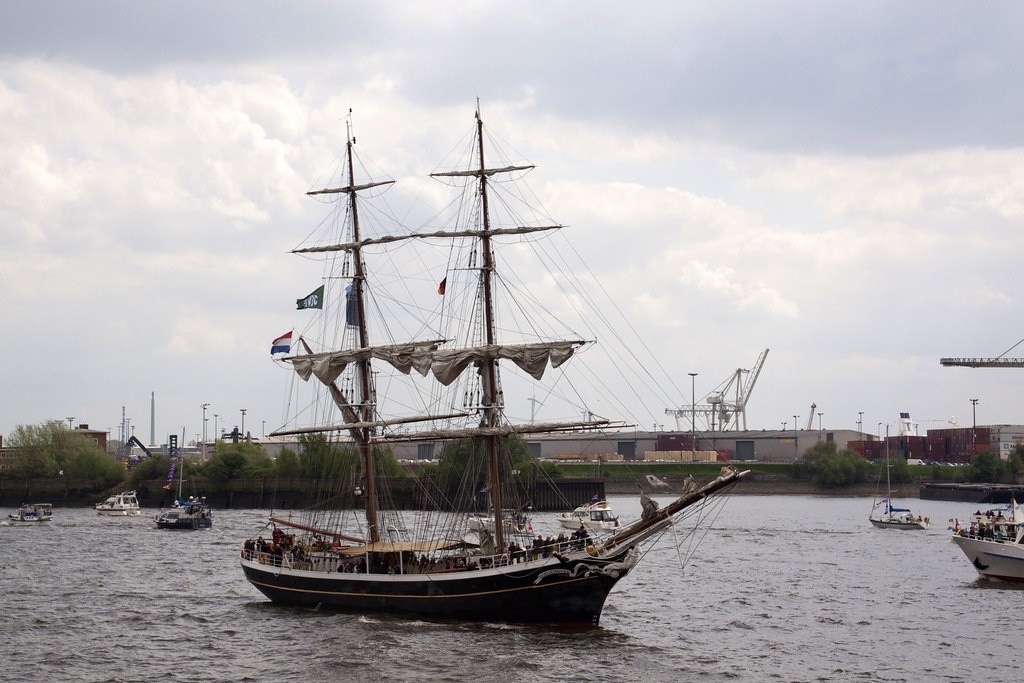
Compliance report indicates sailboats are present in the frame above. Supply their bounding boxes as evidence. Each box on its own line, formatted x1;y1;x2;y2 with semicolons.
240;96;752;631
868;423;932;531
153;425;215;531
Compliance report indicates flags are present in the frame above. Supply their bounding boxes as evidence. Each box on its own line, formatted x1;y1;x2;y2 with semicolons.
437;277;446;295
296;284;324;310
270;331;292;355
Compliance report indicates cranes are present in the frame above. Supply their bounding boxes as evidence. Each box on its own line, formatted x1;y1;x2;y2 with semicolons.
806;402;817;431
664;347;770;431
940;338;1024;368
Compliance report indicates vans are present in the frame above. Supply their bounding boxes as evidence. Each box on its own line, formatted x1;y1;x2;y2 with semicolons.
907;458;927;466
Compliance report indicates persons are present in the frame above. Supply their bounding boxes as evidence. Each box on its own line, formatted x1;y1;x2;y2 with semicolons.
509;526;593;565
244;534;305;567
421;554;435;567
968;510;1006;542
336;562;367;573
186;496;201;515
388;565;408;574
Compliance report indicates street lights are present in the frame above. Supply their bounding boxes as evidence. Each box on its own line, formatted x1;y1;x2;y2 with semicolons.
970;398;979;460
818;413;824;441
214;414;218;439
793;415;801;456
858;411;864;440
118;418;135;446
856;422;862;441
877;422;882;441
108;427;111;450
688;373;699;462
205;418;210;442
196;433;201;442
262;421;266;438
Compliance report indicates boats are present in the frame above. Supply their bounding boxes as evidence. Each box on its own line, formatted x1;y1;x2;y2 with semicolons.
94;487;141;518
948;487;1024;585
7;503;54;527
